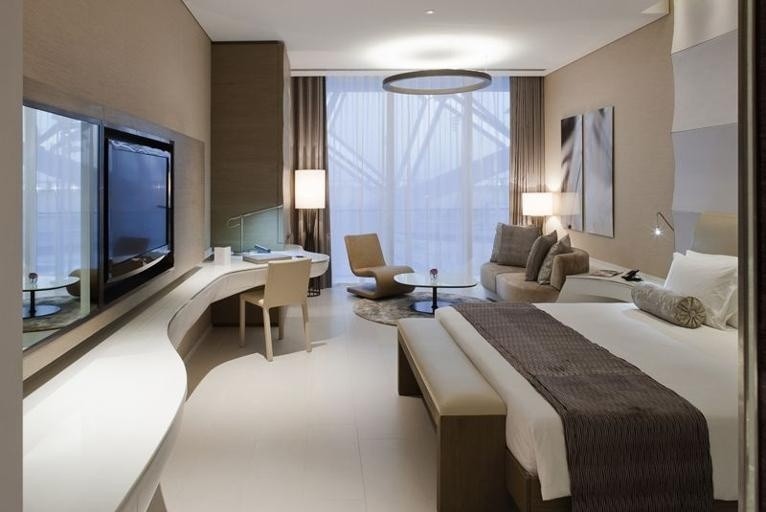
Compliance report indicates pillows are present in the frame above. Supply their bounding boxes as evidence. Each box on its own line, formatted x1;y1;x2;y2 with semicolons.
631;281;707;330
663;251;737;331
686;248;738;330
497;224;543;268
489;223;504;262
523;230;558;282
536;232;571;285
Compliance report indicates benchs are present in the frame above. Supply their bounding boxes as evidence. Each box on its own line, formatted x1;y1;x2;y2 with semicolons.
396;316;509;511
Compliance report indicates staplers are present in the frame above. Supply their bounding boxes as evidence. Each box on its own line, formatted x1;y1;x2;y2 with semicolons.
255;244;270;253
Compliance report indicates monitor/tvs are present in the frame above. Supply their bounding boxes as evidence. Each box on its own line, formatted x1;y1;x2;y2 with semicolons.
100;118;175;307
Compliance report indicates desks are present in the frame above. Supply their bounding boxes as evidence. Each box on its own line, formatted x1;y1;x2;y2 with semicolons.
21;274;81;319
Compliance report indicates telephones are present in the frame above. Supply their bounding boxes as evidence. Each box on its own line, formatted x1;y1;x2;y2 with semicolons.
622;270;642;281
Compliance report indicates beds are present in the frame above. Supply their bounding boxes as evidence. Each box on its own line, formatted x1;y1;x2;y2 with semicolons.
433;207;739;512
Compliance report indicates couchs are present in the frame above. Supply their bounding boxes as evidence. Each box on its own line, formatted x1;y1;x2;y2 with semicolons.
480;247;589;303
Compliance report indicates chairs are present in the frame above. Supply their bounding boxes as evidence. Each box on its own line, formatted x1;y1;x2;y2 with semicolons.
344;233;415;300
238;258;312;362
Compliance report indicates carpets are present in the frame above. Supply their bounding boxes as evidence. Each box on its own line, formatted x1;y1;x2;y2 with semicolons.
353;291;492;326
21;296;99;334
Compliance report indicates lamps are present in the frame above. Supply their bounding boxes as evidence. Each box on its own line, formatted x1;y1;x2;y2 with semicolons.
294;169;325;298
